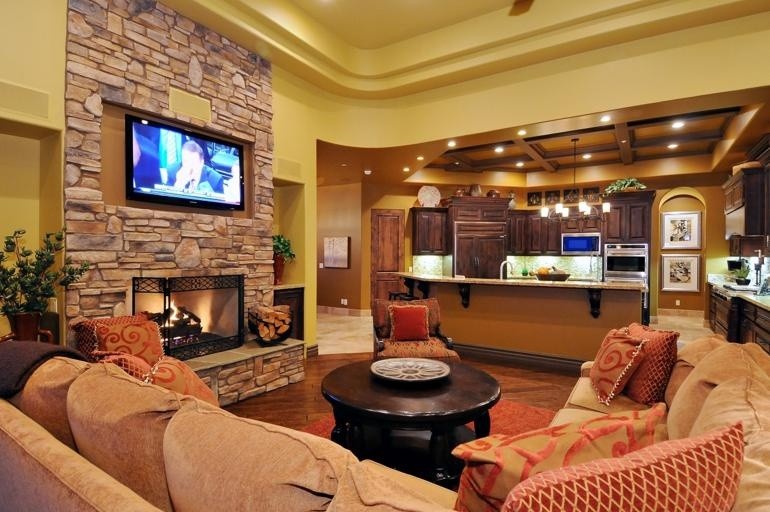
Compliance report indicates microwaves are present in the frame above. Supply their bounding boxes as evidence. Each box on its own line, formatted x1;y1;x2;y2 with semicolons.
560;232;601;256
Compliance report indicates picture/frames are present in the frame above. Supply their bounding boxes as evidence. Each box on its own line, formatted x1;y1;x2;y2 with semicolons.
660;211;701;250
660;254;700;292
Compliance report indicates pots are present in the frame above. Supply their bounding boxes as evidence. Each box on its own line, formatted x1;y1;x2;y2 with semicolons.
728;261;747;271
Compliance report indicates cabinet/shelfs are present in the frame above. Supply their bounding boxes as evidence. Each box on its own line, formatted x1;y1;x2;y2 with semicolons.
722;166;765;239
410;191;655;279
730;235;743;257
274;286;304;342
708;286;770;355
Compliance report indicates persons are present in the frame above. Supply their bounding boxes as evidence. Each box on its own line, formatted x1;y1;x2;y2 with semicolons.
169;140;223;195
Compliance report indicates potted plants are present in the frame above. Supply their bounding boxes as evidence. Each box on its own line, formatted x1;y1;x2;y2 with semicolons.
0;225;91;342
272;234;296;285
733;265;751;286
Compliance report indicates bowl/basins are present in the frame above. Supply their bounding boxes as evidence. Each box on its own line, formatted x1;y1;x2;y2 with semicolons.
736;278;750;285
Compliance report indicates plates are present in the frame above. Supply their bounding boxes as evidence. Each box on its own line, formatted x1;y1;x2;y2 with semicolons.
417;186;441;208
537;274;569;281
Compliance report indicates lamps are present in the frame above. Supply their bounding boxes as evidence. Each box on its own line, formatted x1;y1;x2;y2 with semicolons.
541;138;611;222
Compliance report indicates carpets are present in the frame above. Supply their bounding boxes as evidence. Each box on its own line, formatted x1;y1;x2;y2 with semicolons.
304;402;557;440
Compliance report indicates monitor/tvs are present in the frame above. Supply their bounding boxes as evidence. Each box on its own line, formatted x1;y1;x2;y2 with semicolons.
124;113;245;212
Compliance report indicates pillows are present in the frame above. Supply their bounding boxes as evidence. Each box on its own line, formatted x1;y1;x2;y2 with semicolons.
388;304;429;342
70;311;220;408
590;322;680;405
451;402;668;512
502;419;743;512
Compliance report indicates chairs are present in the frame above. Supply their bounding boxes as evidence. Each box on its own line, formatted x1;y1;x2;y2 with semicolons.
371;298;459;360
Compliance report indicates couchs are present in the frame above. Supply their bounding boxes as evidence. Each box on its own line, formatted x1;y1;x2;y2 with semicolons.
0;339;458;512
549;334;770;512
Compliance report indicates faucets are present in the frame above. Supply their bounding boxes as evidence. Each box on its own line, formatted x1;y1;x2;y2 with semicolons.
500;260;513;280
589;251;599;283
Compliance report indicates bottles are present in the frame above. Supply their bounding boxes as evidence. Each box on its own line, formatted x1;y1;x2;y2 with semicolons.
522;266;529;276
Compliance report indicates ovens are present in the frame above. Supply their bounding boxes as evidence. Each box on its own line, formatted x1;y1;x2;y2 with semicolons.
604;244;648;309
